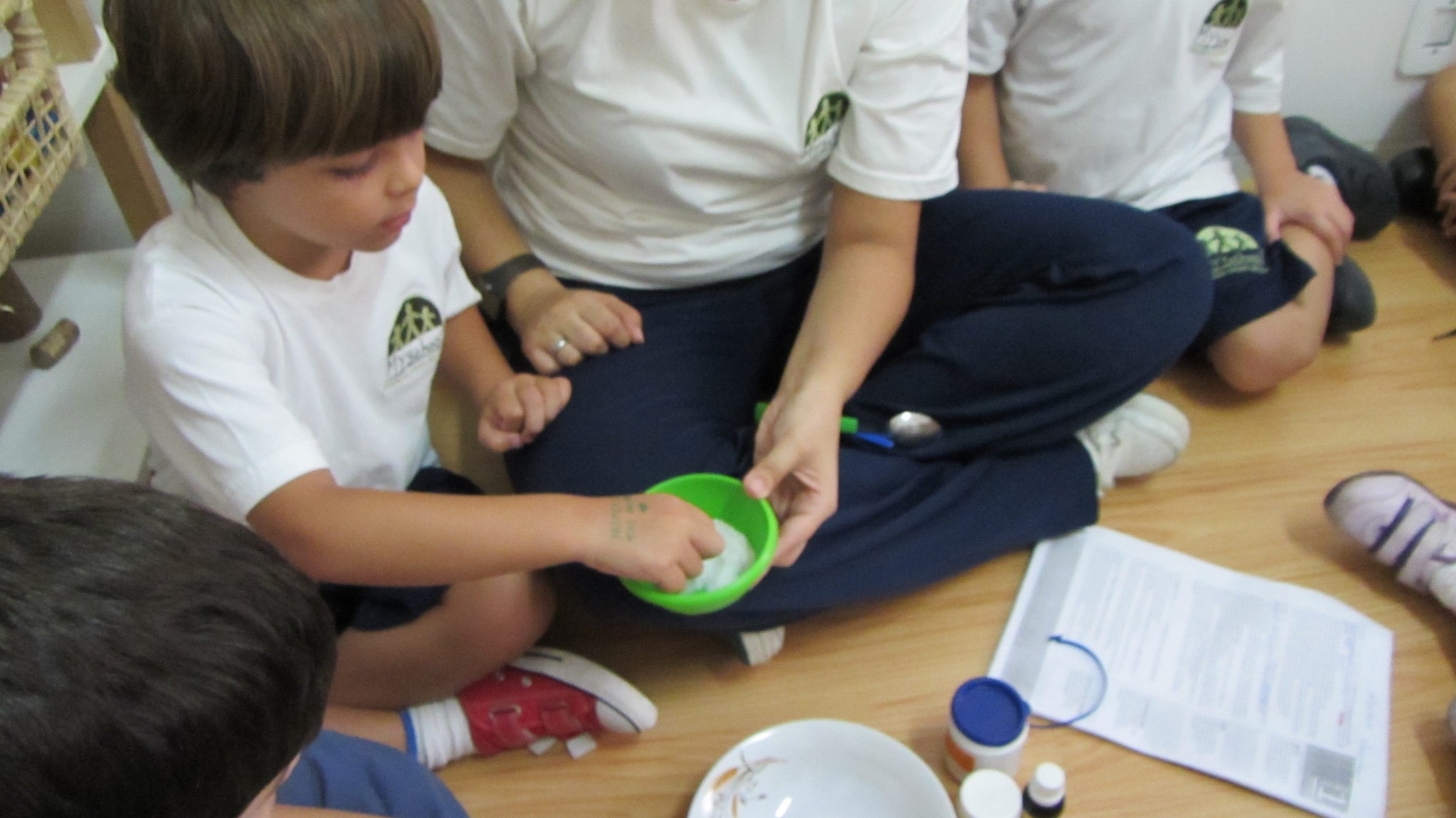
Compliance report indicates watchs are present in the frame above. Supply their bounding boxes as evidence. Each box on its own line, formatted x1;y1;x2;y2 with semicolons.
471;253;550;321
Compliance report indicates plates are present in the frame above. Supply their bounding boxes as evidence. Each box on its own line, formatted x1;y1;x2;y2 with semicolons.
686;718;957;817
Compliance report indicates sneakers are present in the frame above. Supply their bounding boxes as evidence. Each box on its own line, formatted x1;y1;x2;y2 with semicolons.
457;646;658;758
1328;253;1377;332
1283;116;1397;241
1322;470;1456;616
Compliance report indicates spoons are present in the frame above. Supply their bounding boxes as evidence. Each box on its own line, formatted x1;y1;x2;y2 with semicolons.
756;398;944;448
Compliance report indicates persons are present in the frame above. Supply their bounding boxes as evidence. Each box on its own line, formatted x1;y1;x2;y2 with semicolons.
1;470;471;818
412;0;1216;669
956;0;1398;392
100;0;661;773
1387;0;1456;245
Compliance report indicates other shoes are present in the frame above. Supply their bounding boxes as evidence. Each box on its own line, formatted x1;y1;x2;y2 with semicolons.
736;624;786;666
1388;147;1442;227
1074;392;1192;497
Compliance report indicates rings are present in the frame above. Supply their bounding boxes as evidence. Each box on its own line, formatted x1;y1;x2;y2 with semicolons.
548;337;568;354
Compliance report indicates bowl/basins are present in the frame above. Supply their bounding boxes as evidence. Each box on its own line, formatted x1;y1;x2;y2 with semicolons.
619;473;777;615
